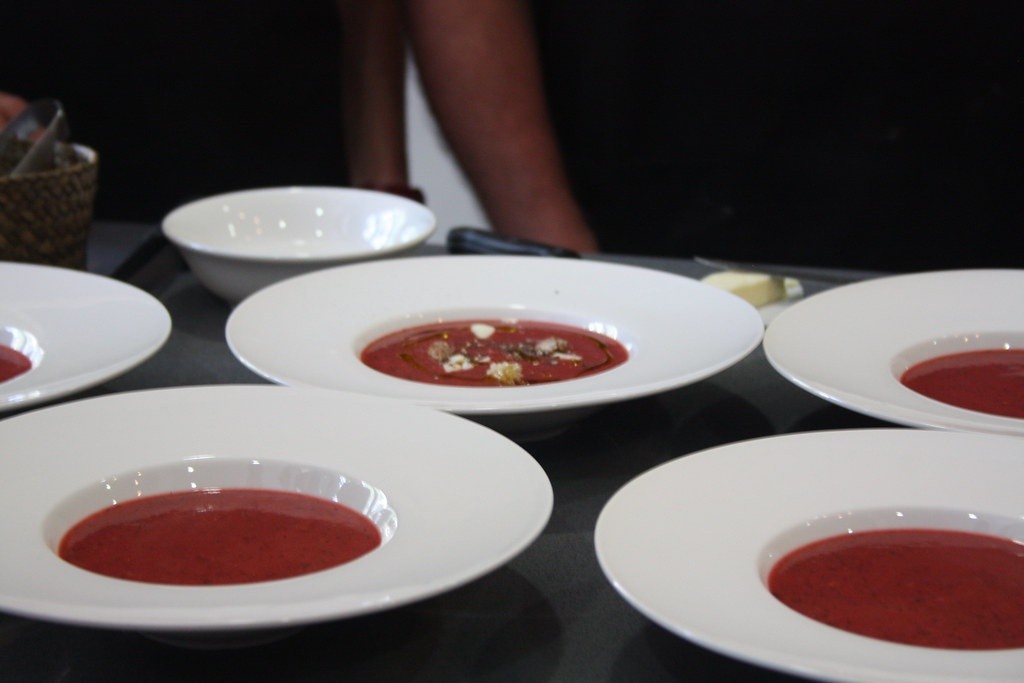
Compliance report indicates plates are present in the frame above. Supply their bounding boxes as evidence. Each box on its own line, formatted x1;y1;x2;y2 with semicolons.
225;255;762;414
593;430;1024;683
0;383;552;633
763;269;1024;435
0;262;174;413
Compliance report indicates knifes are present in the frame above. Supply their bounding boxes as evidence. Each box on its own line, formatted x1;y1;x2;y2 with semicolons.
448;224;775;296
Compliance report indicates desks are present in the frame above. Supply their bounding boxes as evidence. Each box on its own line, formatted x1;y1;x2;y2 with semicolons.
0;217;904;683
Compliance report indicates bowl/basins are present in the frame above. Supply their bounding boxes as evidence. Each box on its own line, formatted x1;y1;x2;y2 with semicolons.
159;186;440;301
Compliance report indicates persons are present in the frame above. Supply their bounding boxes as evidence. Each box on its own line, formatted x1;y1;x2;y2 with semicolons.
0;0;406;226
403;0;1024;271
